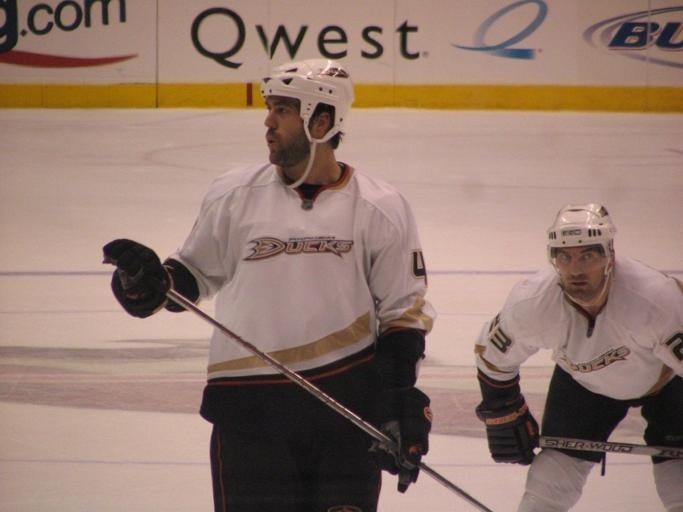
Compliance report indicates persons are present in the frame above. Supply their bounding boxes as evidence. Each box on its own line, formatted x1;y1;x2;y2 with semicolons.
102;58;437;512
473;204;683;511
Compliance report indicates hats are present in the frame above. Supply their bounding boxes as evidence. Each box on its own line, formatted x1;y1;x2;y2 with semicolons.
263;58;355;143
545;201;619;276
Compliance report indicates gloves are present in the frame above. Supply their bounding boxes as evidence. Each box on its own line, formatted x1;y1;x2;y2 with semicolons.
102;239;167;319
366;385;433;494
476;394;539;466
